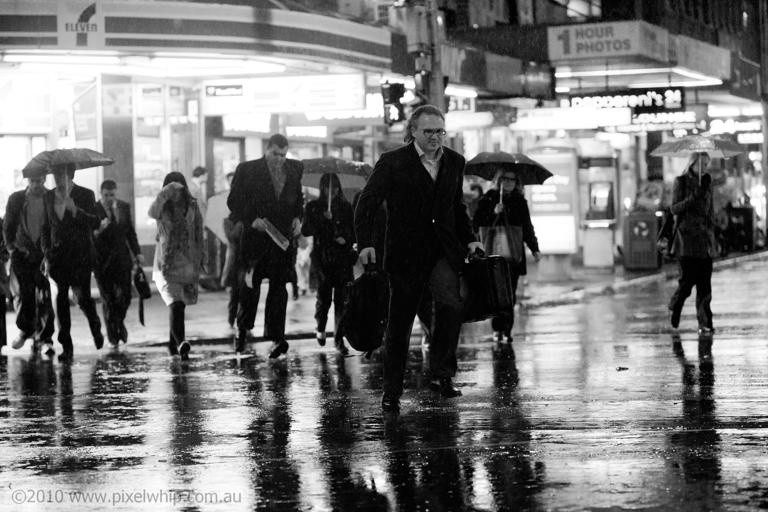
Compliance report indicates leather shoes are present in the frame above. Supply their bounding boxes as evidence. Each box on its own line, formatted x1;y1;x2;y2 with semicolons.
428;377;463;400
381;391;402;412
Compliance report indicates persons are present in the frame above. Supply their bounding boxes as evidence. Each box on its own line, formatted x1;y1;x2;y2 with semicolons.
147;170;206;360
352;105;488;413
40;165;105;363
226;133;306;362
1;171;57;356
473;167;543;346
91;178;146;346
667;151;720;334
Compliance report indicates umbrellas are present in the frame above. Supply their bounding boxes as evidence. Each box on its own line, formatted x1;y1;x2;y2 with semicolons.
648;132;744;188
299;157;373;213
22;147;116;198
463;149;555;205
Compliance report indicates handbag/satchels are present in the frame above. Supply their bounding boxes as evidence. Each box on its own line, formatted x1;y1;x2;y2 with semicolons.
130;259;153;301
337;253;391;353
478;207;525;272
655;225;692;264
334;218;360;266
458;247;517;325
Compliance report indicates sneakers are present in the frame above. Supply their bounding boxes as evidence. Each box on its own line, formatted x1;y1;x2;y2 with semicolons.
670;310;681;328
697;326;716;334
332;340;349;354
0;326;79;364
233;329;248;353
89;325;132;351
177;340;191;364
493;332;515;344
268;339;290;361
315;329;328;348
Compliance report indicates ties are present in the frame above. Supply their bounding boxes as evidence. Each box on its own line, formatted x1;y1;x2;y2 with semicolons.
107;205;117;227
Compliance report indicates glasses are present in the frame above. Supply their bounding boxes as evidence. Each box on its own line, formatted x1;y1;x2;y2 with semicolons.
500;176;517;184
415;127;447;139
